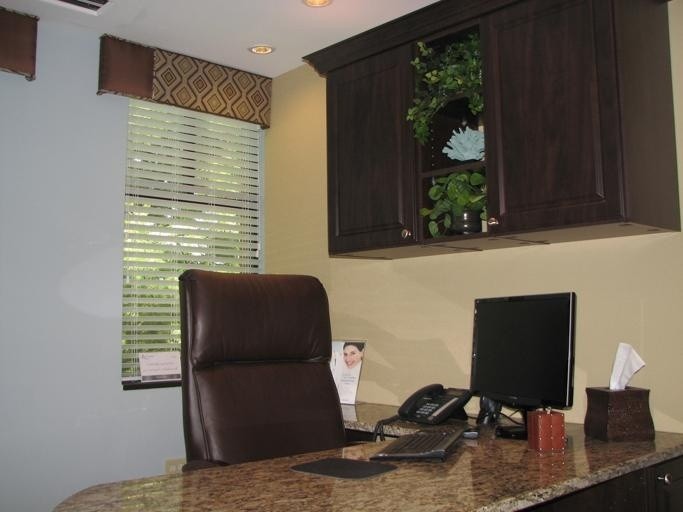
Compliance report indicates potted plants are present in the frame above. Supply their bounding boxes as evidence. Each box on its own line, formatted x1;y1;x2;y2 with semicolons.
418;171;488;238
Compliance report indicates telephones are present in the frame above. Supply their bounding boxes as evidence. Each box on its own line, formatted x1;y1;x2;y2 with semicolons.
398;383;473;426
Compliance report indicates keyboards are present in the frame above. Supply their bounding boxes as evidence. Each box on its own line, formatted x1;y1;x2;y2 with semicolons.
371;424;466;462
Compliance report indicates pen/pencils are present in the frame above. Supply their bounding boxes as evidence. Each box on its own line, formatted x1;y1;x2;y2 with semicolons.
547;406;551;414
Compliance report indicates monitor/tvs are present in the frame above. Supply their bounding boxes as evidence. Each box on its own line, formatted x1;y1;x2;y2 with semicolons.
468;290;576;439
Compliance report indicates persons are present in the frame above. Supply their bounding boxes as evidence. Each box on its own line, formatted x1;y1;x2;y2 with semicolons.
341;341;364;368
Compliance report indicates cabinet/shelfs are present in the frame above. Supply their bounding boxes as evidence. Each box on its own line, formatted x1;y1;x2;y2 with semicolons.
492;1;626;229
651;457;682;512
415;20;489;243
325;42;414;262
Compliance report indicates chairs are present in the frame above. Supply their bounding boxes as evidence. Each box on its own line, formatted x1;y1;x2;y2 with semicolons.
178;269;368;472
